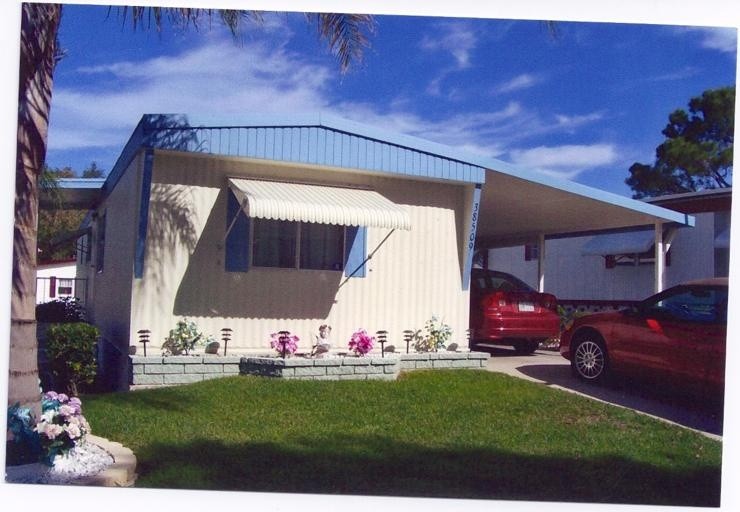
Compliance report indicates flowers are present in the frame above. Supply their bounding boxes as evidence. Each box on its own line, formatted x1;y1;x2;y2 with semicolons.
348;328;373;355
173;318;204;353
271;333;298;354
15;391;90;464
424;317;451;352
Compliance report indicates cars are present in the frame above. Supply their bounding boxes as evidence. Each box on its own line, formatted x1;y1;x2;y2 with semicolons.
561;277;729;402
469;268;561;356
